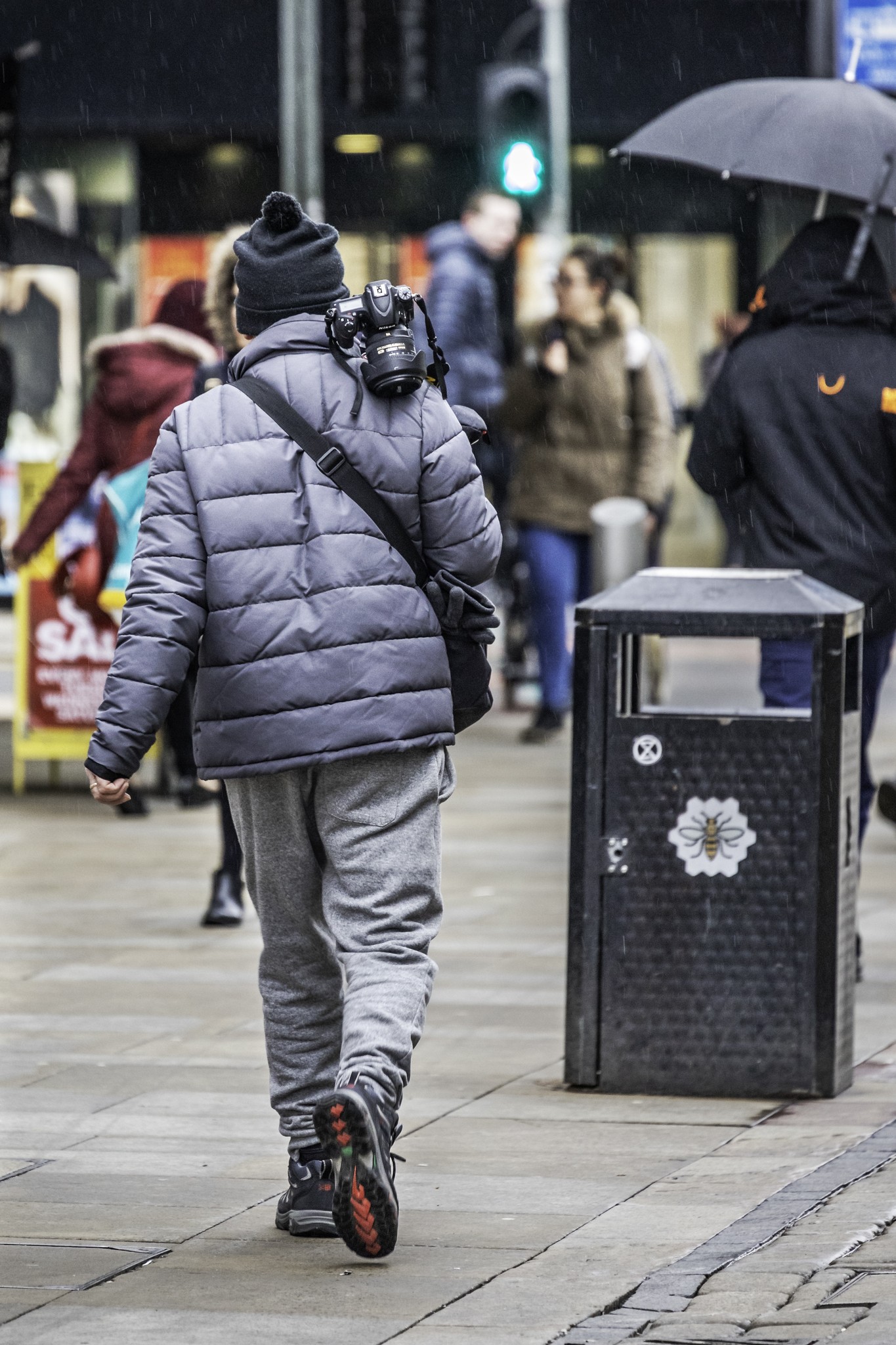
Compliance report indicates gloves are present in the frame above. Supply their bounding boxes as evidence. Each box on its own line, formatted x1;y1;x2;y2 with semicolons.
425;570;502;643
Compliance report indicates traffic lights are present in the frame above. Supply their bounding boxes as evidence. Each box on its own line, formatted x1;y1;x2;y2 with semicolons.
480;63;558;236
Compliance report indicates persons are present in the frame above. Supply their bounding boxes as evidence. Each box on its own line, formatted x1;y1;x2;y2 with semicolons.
183;222;246;928
10;280;222;632
684;213;896;980
491;245;675;745
416;188;523;509
83;191;504;1258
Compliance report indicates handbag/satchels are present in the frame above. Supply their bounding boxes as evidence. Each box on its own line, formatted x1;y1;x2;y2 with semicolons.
441;629;492;733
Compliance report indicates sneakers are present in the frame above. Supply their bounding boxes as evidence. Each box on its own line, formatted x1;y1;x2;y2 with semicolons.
313;1085;404;1258
274;1154;341;1237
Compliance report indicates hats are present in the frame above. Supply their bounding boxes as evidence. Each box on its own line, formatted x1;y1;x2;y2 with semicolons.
231;189;348;334
158;281;217;342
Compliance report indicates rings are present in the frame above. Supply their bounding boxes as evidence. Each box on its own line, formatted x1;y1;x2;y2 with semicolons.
90;783;97;789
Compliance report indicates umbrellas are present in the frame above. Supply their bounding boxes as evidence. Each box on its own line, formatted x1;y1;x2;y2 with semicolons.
607;38;896;219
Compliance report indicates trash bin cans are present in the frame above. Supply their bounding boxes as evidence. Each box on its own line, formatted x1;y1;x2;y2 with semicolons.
560;566;862;1099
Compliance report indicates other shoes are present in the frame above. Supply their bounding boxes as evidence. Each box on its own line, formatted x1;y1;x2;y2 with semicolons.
521;709;563;745
203;869;244;924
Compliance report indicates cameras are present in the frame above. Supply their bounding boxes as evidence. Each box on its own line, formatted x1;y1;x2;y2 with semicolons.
330;278;427;399
